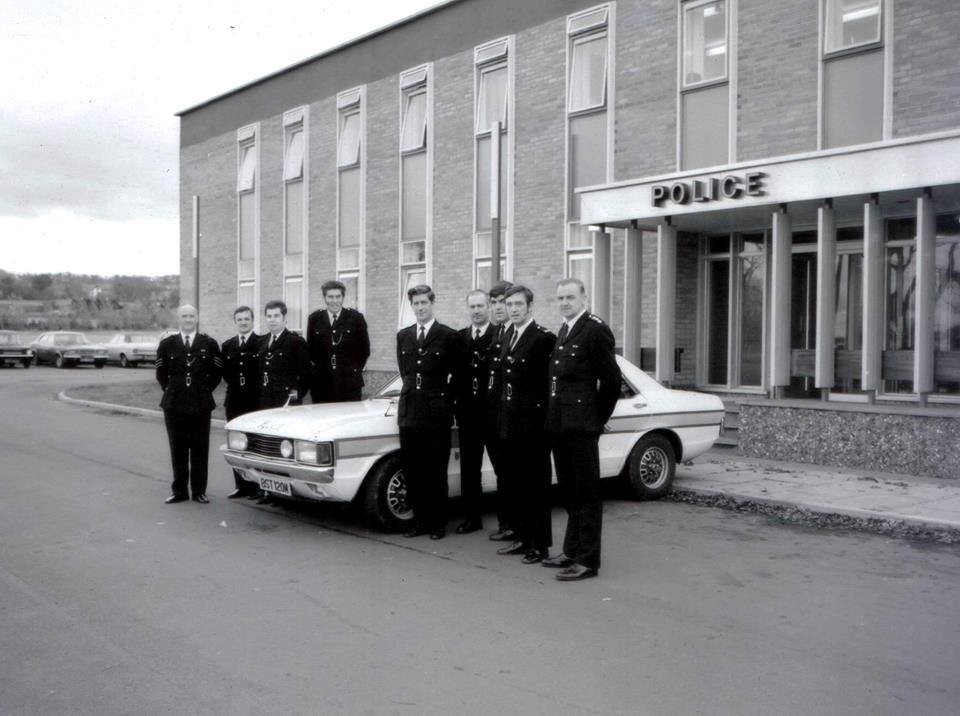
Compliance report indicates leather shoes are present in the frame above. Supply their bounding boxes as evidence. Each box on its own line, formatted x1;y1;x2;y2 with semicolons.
522;545;550;564
490;528;516;540
165;494;190;504
430;531;445;539
403;527;429;537
191;494;210;504
229;489;279;506
496;541;529;555
542;551;577;567
557;559;596;580
456;520;484;533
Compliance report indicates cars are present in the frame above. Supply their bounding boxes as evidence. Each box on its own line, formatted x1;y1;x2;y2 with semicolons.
97;333;160;368
27;331;109;369
219;353;728;536
0;330;35;368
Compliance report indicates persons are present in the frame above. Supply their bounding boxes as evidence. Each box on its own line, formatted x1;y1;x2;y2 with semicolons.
496;285;556;563
489;281;512;540
308;280;371;404
219;306;266;500
533;277;622;579
454;289;496;534
397;284;465;540
257;301;307;507
157;304;222;504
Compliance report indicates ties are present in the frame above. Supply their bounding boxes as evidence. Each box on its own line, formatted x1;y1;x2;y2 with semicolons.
184;335;191;350
559;324;569;343
474;328;481;339
509;330;517;347
332;313;337;325
418;326;426;348
240;336;246;348
500;325;506;337
270;335;277;348
